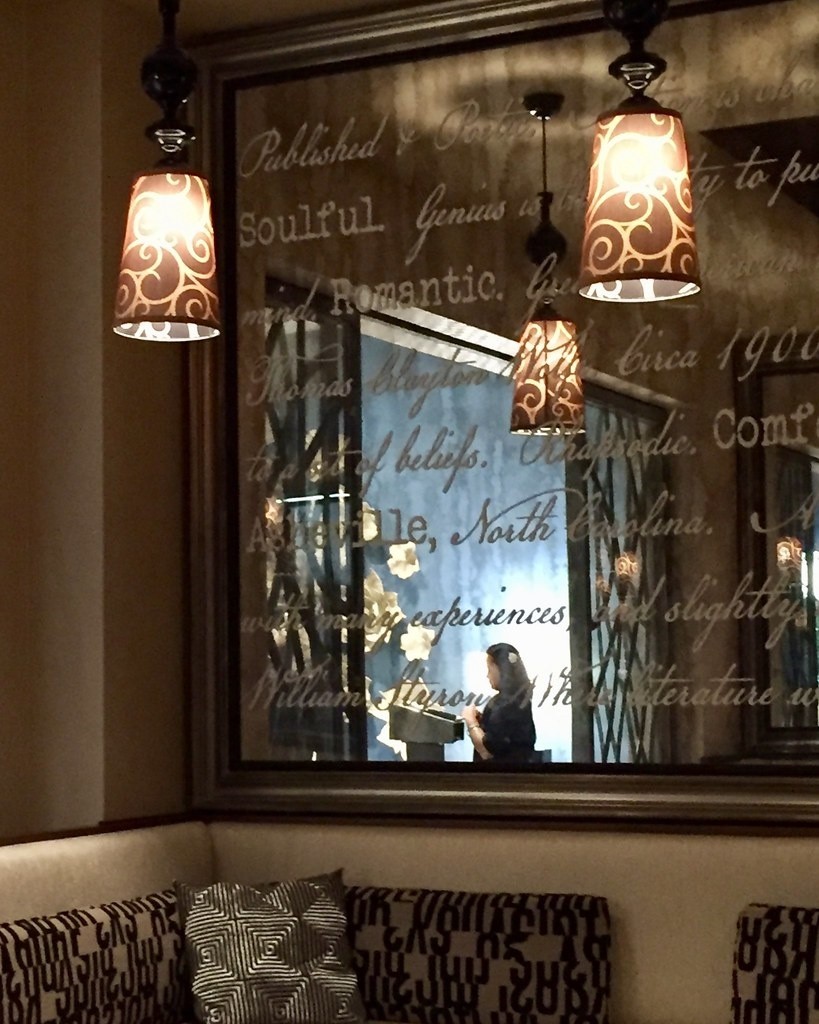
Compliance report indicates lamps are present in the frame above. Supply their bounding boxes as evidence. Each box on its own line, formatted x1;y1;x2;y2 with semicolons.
509;90;586;435
111;1;224;343
568;0;702;306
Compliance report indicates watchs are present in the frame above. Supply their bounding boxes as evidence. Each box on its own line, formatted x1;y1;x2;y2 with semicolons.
468;723;481;732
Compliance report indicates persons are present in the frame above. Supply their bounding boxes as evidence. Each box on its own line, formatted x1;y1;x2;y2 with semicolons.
460;643;537;763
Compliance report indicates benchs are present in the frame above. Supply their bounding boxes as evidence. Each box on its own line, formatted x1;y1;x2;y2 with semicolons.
0;808;818;1024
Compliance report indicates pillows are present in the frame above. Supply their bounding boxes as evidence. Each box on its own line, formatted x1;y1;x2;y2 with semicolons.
171;867;369;1024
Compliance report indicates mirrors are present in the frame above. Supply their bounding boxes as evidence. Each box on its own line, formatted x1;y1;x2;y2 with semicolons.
188;0;819;835
